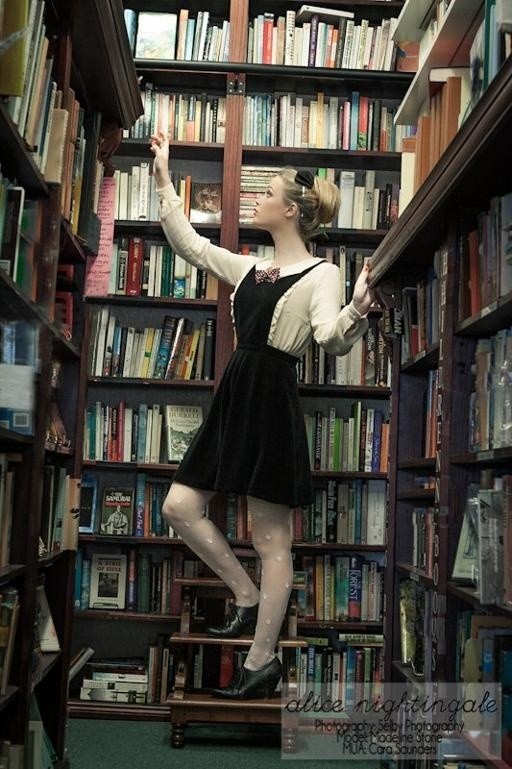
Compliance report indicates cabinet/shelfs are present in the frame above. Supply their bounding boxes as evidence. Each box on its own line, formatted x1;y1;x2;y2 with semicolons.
0;0;512;769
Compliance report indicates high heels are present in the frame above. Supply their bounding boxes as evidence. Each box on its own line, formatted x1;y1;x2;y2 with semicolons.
207;602;259;639
209;656;281;699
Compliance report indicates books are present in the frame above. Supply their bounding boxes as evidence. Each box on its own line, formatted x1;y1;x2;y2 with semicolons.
0;0;512;767
